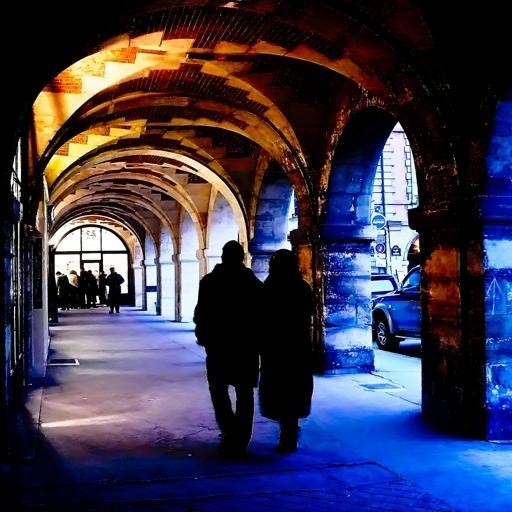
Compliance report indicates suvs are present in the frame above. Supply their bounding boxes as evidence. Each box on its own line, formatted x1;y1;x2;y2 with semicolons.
371;263;421;349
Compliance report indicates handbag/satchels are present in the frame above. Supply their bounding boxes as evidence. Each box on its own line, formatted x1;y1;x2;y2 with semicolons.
309;307;327;376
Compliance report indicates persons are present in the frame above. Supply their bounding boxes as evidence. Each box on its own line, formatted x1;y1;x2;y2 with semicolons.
259;249;319;454
105;268;125;315
57;266;109;312
193;240;272;465
